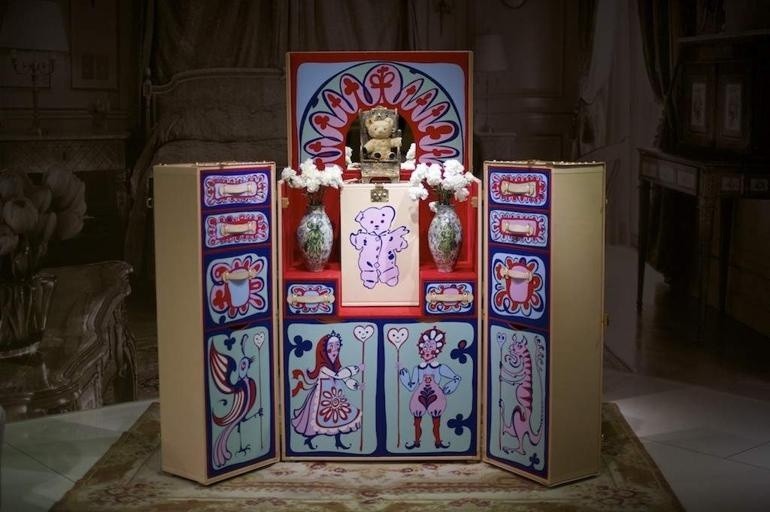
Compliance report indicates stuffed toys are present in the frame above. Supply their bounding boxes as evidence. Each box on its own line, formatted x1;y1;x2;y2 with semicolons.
364;117;403;160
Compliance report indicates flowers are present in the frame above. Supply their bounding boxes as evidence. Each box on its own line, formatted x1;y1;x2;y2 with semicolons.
0;159;87;280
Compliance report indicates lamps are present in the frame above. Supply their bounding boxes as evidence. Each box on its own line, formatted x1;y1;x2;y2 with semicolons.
0;0;69;137
473;34;506;133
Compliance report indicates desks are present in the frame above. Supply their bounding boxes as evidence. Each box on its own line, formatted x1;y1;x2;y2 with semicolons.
636;29;769;350
0;260;139;422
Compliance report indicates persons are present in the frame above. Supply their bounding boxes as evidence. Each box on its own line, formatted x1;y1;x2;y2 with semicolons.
291;332;367;451
396;327;461;449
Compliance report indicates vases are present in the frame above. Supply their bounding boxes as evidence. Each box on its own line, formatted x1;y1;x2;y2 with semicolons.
0;270;58;359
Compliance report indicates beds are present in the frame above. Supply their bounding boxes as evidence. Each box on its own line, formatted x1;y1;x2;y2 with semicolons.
143;66;286;283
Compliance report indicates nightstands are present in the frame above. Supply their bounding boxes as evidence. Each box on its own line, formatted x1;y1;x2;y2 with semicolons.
477;135;561;174
0;131;131;219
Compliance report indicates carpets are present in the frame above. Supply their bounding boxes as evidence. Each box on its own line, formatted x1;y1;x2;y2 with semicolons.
49;402;686;512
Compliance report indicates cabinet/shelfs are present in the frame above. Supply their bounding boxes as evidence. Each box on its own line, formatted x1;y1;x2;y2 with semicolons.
153;51;605;488
